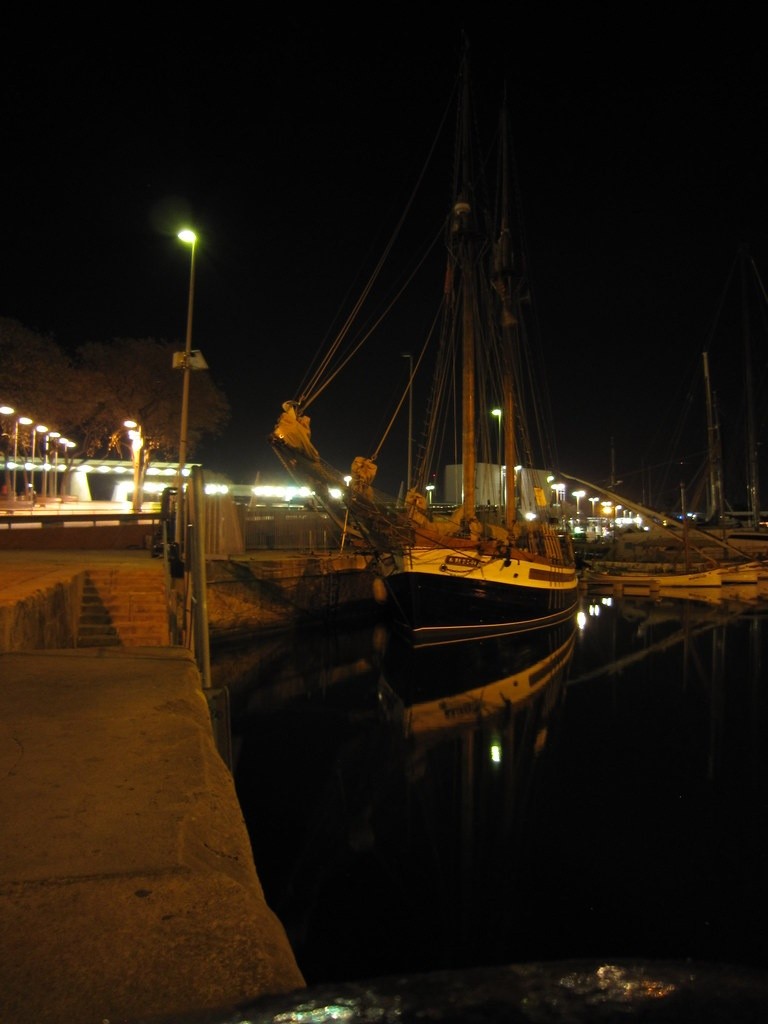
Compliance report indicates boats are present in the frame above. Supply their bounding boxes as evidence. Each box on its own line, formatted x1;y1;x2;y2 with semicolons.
709;554;761;586
581;561;726;591
751;553;768;580
329;624;596;825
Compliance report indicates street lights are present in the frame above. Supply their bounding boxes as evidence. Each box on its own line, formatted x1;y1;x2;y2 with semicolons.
42;431;63;500
589;498;600;517
173;230;199;557
492;409;502;521
12;417;33;501
614;506;624;522
342;475;353;489
64;440;78;493
571;490;585;516
551;483;565;521
425;485;435;506
54;437;70;498
501;465;507;510
29;426;49;496
122;420;143;513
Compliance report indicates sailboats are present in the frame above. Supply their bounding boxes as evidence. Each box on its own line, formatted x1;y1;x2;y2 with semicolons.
268;142;592;646
